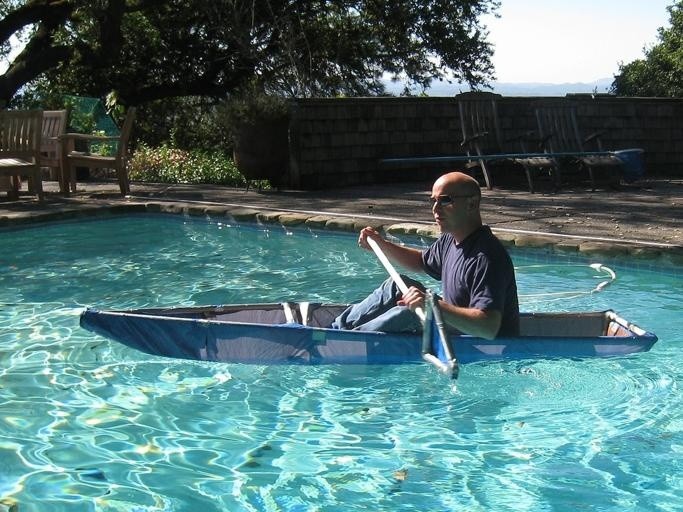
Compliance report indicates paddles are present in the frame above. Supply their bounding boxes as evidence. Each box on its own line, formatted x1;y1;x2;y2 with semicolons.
367;235;459;380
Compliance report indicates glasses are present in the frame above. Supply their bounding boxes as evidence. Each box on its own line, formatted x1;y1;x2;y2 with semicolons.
430;195;472;207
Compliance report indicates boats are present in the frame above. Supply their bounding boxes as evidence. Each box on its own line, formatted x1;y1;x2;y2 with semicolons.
74;302;659;365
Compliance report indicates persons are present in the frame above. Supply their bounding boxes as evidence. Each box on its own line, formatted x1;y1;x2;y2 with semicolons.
325;171;520;340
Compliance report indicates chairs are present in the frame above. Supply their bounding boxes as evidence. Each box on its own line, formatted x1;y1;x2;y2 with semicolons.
0;105;139;201
534;104;626;192
455;91;556;193
